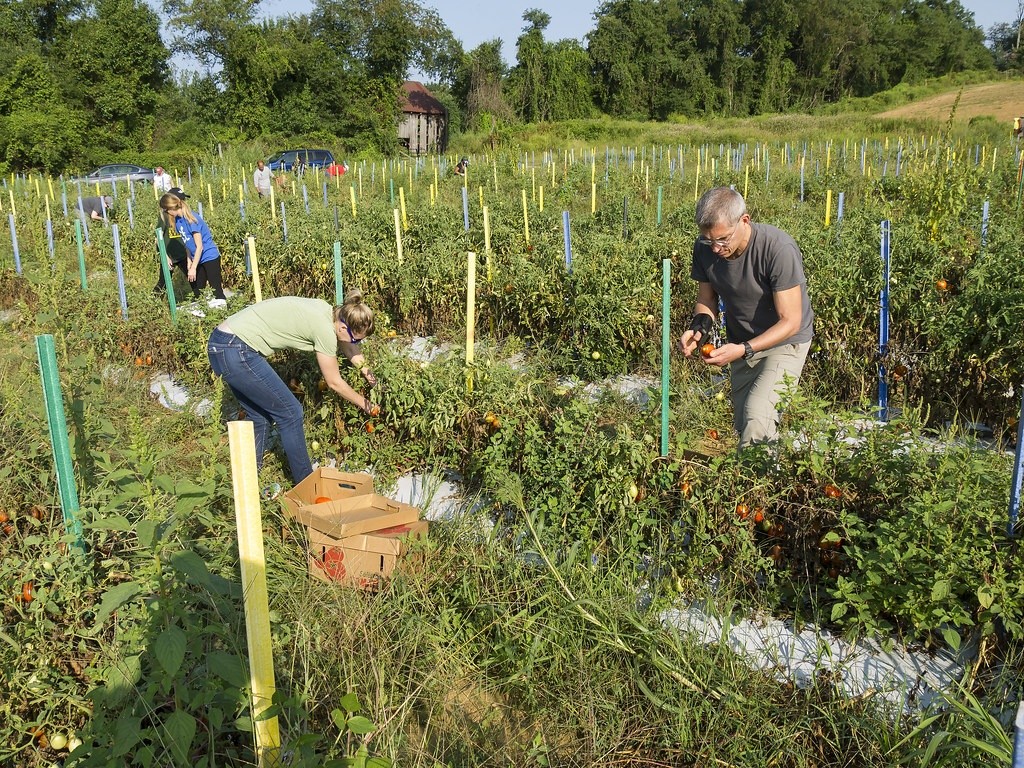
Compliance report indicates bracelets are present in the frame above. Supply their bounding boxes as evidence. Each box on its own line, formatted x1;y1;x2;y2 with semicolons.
357;365;364;371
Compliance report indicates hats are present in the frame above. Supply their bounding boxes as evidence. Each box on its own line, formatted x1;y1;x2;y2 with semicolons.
105;196;114;209
461;156;469;163
168;188;191;200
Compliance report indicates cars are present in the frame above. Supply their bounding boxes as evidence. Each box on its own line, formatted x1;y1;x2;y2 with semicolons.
66;164;157;184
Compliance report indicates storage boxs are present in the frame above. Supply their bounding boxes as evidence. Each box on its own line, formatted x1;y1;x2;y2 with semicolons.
279;467;431;592
276;176;285;190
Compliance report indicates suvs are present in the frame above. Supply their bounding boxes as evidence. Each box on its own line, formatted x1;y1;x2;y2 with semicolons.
266;150;334;171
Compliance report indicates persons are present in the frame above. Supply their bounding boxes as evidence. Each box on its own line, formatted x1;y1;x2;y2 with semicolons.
154;167;174;192
292;155;305;179
151;187;226;316
254;161;280;199
207;289;381;485
678;188;814;485
454;156;469;176
74;196;114;230
325;164;349;178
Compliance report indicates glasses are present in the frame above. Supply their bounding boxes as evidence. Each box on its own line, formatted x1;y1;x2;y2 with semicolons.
341;319;361;343
165;209;168;213
698;214;744;246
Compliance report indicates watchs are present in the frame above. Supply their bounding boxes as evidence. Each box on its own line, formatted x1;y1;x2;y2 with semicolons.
740;342;755;360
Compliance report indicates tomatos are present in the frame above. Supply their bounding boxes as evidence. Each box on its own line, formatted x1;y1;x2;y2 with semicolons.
315;497;332;505
702;344;715;357
680;427;847;580
0;508;43;602
313;377;503;452
937;280;947;290
118;341;153;366
